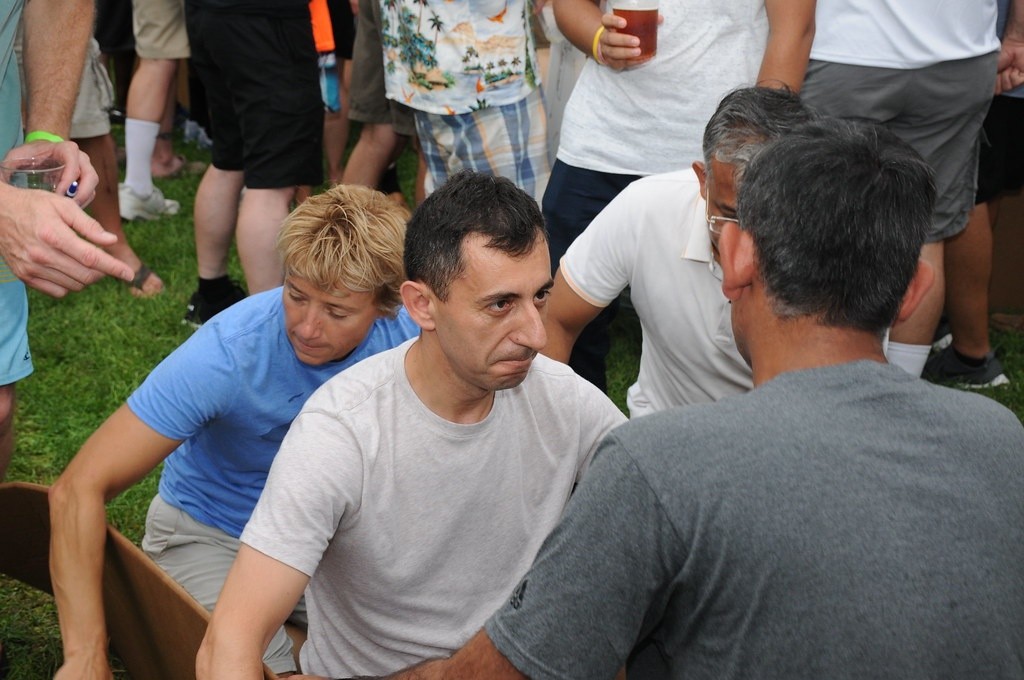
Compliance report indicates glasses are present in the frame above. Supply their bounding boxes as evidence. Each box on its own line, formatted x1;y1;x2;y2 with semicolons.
704;174;741;233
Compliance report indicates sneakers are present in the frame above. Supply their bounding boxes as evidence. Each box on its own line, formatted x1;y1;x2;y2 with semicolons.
922;330;1008;390
117;183;179;220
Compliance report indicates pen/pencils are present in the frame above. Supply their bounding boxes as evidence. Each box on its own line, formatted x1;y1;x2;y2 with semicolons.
64;181;78;198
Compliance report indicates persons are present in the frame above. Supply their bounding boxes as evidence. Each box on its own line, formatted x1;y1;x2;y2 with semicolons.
194;172;635;680
47;185;421;680
281;118;1024;680
1;0;1024;481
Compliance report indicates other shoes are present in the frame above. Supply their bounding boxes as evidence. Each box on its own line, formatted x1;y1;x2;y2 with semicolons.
182;282;246;329
151;155;206;181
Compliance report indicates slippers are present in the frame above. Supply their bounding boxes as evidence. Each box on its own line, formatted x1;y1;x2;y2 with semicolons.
112;263;164;299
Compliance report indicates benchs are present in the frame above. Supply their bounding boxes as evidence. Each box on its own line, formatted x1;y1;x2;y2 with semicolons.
0;480;309;680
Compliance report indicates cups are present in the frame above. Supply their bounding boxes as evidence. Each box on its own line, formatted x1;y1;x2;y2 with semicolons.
0;157;66;192
611;0;659;59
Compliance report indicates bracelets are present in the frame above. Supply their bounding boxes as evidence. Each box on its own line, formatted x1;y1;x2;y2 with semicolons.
592;26;606;65
24;131;63;143
